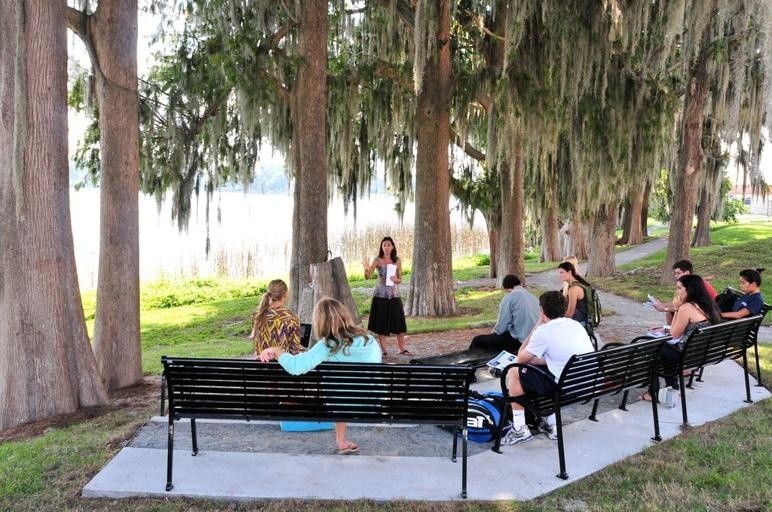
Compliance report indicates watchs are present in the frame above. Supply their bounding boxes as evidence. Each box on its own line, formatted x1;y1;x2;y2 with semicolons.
663;304;668;312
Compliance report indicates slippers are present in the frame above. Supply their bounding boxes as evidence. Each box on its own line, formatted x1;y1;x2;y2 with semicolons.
399;350;411;355
636;391;655;402
338;442;359;455
383;352;389;358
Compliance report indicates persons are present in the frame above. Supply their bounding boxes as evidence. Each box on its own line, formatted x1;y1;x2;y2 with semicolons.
558;262;594;338
714;269;763;350
360;236;413;360
637;274;722;402
560;255;582;306
652;258;719;334
501;291;606;446
248;279;305;355
259;299;382;456
469;274;540;351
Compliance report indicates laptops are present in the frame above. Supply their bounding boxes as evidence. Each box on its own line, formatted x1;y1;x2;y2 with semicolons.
298;323;313;351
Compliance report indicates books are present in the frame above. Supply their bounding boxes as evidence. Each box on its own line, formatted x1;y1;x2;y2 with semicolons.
487;350;518;371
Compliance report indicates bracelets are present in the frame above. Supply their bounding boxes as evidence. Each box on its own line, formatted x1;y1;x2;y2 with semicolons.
723;313;726;317
674;309;678;312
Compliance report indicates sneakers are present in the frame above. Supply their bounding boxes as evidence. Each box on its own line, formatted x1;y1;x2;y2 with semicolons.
500;420;534;447
538;422;559;441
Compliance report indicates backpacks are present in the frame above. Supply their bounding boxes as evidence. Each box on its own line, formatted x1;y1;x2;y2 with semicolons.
715;287;738;314
566;281;602;332
440;386;506;444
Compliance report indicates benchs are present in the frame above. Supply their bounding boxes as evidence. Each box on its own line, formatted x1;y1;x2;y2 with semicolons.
694;280;772;392
161;353;472;502
491;335;674;480
409;347;499;368
628;313;765;437
